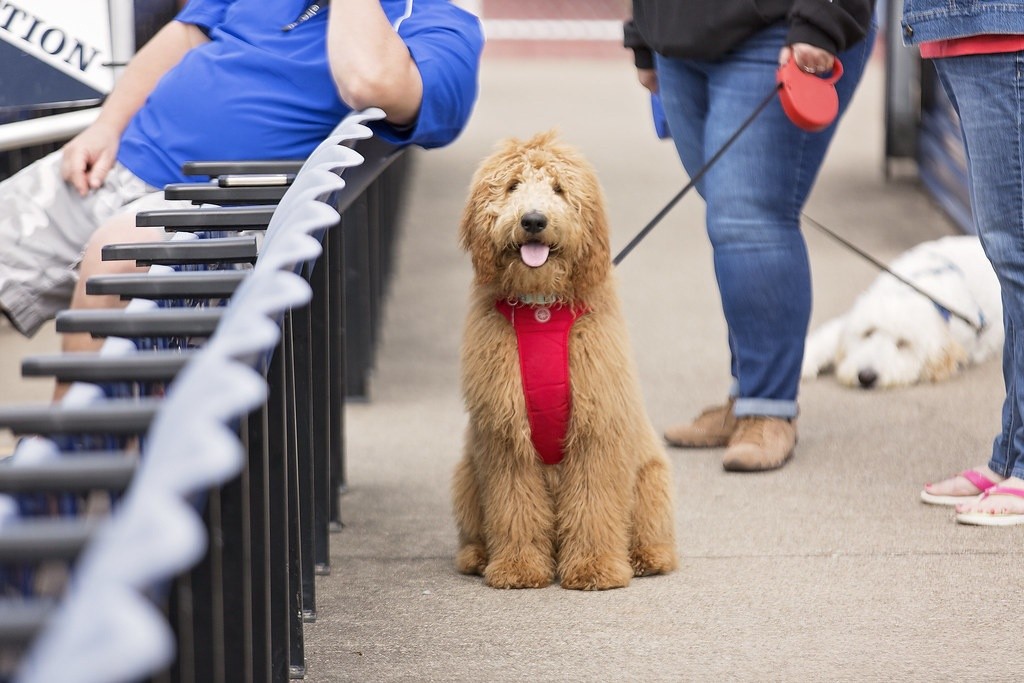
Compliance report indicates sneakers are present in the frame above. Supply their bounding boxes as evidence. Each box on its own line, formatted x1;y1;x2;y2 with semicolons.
723;415;796;471
664;405;738;448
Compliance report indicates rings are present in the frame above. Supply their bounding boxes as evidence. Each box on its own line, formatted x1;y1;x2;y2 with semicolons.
814;65;826;72
804;64;816;73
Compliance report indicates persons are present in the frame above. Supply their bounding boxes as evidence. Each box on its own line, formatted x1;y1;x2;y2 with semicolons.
902;0;1024;526
0;0;486;403
623;0;878;471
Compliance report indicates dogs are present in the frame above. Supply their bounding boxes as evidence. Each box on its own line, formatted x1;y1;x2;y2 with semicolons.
797;232;1005;389
451;134;678;589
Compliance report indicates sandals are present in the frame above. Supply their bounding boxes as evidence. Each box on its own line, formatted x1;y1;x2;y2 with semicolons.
957;484;1024;525
921;467;996;506
